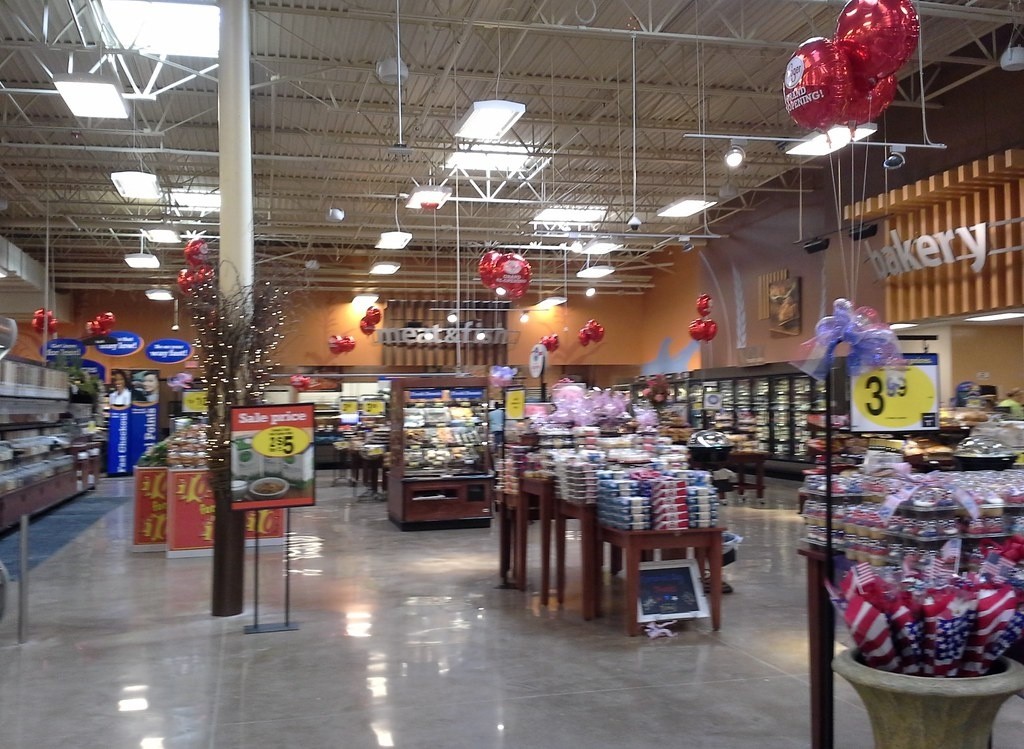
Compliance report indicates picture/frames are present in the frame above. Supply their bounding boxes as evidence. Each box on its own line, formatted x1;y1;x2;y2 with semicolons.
637;559;711;624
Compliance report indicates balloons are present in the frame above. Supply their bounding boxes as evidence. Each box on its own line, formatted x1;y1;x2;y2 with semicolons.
328;334;356;355
577;318;605;346
90;311;116;336
539;333;560;353
689;293;718;342
360;305;381;336
289;375;311;392
489;364;518;380
177;238;215;296
478;250;531;299
31;307;59;334
813;298;902;373
782;0;921;134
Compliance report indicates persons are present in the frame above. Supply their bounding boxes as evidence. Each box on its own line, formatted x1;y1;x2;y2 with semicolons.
997;386;1024;420
132;370;160;403
109;370;132;406
488;402;505;445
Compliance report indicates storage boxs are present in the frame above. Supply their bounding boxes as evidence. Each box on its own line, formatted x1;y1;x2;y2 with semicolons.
514;447;720;531
231;434;314;491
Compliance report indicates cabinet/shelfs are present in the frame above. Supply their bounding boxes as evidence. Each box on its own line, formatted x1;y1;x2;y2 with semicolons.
615;366;724;446
389;378;495;533
0;355;101;533
795;410;1024;570
725;359;1024;749
313;401;389;493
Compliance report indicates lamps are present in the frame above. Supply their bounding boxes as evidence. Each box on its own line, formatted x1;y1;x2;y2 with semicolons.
144;275;174;301
803;236;831;254
1000;20;1024;71
576;254;615;279
110;152;163;199
451;27;527;144
581;239;625;254
53;24;130;119
723;147;747;167
374;199;412;250
657;195;719;218
535;297;568;309
124;238;161;269
404;169;452;210
351;293;380;304
446;312;457;322
784;121;877;157
141;228;182;244
369;261;402;275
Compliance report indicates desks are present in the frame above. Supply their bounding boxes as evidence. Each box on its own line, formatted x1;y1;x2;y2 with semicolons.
796;548;835;749
